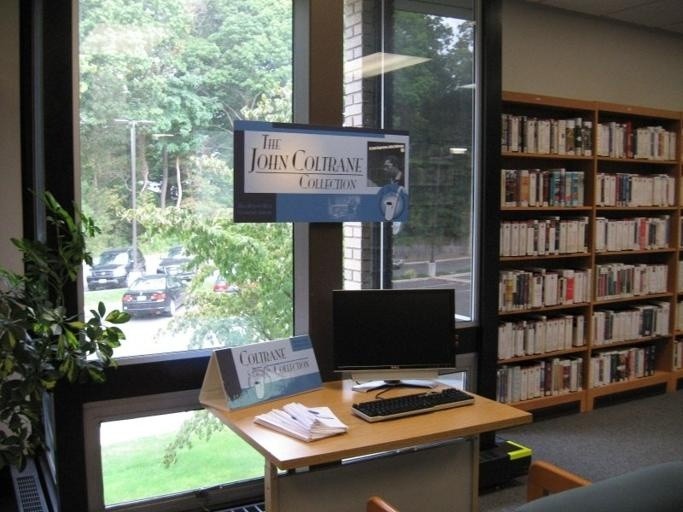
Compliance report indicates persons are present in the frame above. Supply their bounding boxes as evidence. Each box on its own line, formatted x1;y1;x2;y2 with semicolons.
385;154;404;185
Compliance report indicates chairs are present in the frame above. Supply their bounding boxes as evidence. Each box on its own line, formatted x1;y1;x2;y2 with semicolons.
366;460;682;512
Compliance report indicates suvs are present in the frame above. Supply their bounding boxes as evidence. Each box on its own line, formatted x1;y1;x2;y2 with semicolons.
86;248;147;291
157;247;211;282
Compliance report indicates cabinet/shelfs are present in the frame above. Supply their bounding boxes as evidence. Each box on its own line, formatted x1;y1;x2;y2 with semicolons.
496;90;683;414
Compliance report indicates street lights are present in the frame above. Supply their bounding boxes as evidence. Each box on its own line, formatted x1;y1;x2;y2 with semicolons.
112;117;156;272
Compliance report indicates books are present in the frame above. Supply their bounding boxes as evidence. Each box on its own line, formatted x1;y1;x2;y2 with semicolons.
498;313;587;359
500;166;584;207
596;214;672;255
495;356;587;403
500;216;591;257
591;344;671;387
595;263;671;300
593;300;671;346
676;302;683;331
500;113;594;156
673;340;683;370
498;268;592;312
596;171;675;207
252;401;349;443
596;120;680;161
678;261;682;292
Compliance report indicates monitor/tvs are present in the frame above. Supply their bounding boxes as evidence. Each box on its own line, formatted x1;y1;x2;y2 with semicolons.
331;290;455;393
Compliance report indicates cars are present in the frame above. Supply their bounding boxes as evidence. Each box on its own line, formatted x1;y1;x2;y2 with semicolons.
122;275;191;318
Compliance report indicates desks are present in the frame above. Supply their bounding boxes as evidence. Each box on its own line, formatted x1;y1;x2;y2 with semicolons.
204;380;532;512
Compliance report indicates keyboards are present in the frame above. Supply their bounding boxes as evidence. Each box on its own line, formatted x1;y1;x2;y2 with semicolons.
352;389;473;423
